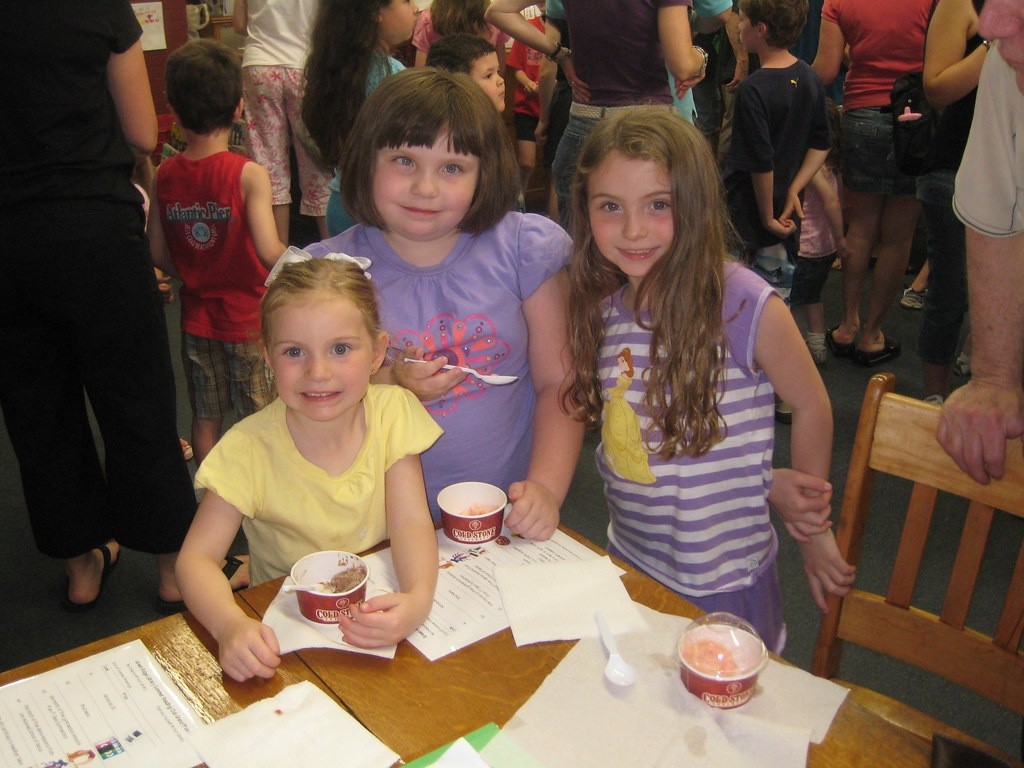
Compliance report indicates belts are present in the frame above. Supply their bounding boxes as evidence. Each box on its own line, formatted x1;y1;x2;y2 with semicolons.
570;103;678;122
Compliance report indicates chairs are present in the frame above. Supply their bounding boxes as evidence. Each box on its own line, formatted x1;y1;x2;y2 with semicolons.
810;373;1024;756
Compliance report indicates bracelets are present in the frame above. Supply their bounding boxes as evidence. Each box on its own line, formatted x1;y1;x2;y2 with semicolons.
548;39;571;64
689;44;709;74
981;39;992;50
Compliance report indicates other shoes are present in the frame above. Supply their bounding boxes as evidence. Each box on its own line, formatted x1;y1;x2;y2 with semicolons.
774;400;793;424
180;437;194;461
901;286;927;309
805;336;827;363
954;357;973;374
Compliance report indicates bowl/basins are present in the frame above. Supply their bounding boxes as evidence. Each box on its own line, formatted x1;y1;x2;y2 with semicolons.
436;482;507;546
291;551;369;626
676;625;768;709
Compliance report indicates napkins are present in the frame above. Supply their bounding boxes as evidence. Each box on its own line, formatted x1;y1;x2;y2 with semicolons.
492;553;646;646
186;680;400;768
264;575;399;660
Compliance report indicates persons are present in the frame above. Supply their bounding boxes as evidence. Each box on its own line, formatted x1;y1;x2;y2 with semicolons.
176;259;447;683
127;0;1024;366
570;106;856;665
285;66;592;543
150;37;323;468
935;0;1024;486
0;0;246;615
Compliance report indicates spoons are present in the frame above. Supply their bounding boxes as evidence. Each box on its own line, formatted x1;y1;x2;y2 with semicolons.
282;584;335;595
403;357;518;386
595;606;637;688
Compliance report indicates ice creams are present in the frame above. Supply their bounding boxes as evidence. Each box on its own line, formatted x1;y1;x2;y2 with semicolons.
328;566;367;594
682;637;741;679
458;504;500;516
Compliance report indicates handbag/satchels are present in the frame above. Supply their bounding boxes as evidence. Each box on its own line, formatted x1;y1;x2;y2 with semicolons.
889;66;937;176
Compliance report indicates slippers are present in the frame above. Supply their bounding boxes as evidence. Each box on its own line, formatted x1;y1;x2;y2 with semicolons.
825;325;857;355
153;552;242;614
63;541;120;614
857;329;902;368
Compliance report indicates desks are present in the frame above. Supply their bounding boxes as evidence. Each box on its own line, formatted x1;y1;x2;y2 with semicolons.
0;501;931;768
